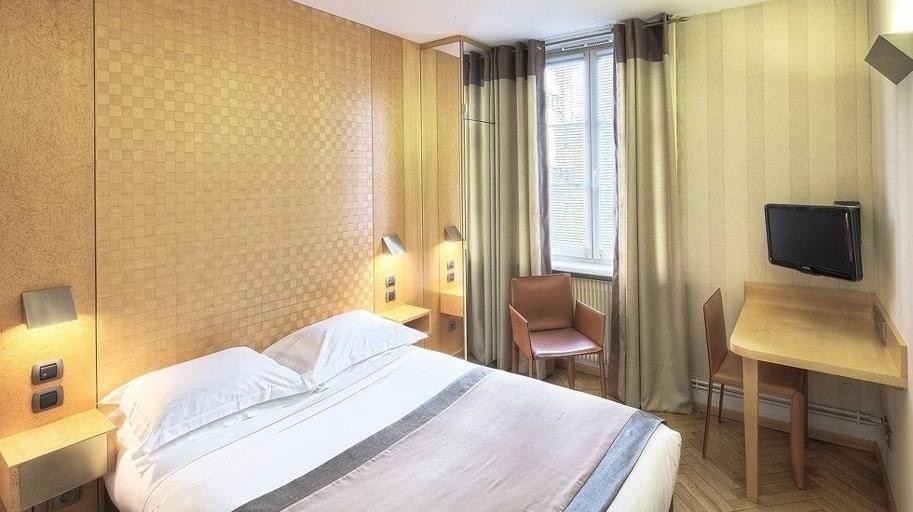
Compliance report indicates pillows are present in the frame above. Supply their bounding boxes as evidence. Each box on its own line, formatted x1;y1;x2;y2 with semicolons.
99;346;329;472
261;308;428;388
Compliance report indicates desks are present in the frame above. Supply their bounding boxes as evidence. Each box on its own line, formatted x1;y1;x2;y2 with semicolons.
729;282;908;503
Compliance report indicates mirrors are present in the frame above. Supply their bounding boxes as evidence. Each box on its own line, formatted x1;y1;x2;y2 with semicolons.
420;38;465;361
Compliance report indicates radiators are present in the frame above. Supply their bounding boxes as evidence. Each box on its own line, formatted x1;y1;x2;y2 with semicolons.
561;277;612;369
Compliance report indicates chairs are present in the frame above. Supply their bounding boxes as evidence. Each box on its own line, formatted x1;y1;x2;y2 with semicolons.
704;289;808;491
511;274;608;400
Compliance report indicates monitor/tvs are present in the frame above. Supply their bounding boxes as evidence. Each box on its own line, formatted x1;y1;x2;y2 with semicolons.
764;202;864;281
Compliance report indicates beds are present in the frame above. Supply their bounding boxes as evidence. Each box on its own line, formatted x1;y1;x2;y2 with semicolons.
98;308;683;512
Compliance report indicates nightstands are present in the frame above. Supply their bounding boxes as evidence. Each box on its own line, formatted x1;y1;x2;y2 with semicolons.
385;304;432;336
440;286;464;318
1;407;119;512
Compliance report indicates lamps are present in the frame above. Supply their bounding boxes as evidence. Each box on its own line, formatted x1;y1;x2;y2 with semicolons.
382;235;407;257
21;285;79;330
446;226;461;242
